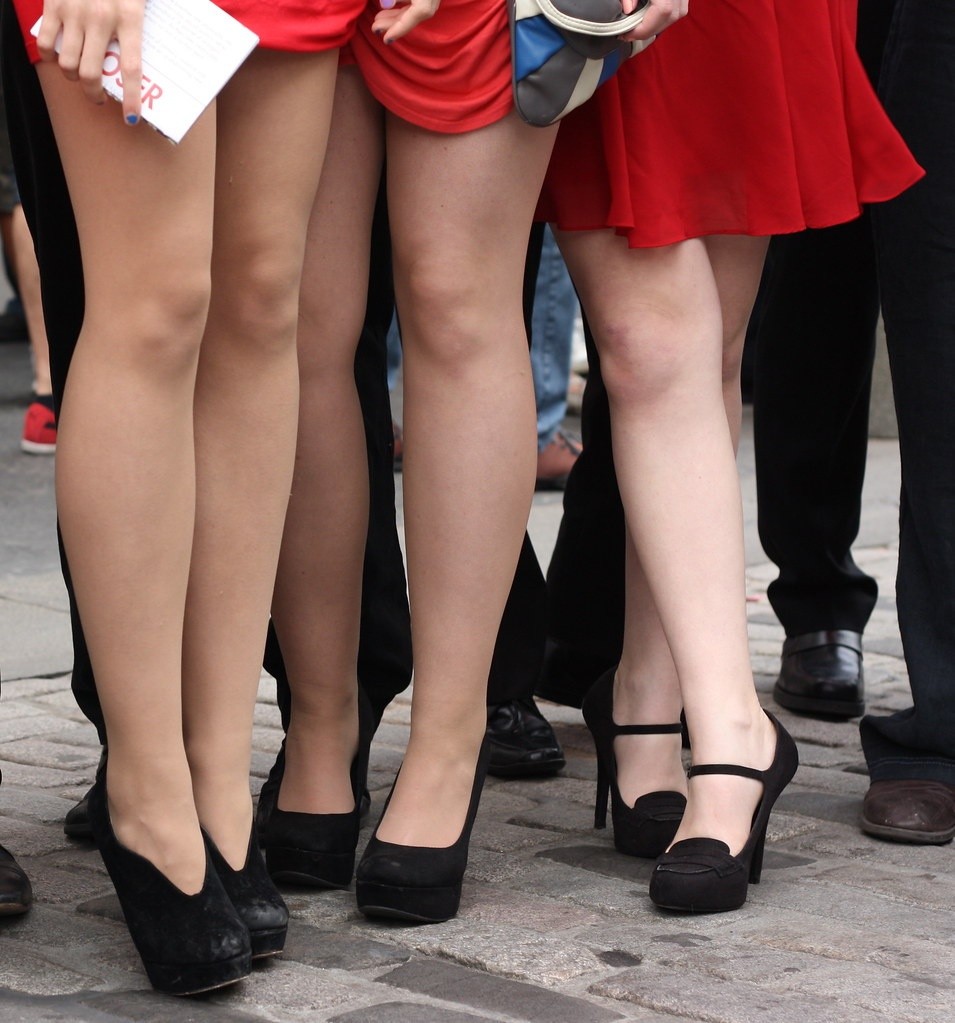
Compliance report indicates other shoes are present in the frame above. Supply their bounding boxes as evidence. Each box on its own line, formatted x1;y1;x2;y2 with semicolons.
0;844;33;913
387;417;405;474
63;784;97;835
18;401;59;453
535;427;582;488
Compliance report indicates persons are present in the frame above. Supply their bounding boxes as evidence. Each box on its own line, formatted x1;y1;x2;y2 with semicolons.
14;0;441;997
533;0;926;912
0;0;955;843
266;0;688;923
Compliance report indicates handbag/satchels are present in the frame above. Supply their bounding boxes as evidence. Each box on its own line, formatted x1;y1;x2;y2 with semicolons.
511;0;659;127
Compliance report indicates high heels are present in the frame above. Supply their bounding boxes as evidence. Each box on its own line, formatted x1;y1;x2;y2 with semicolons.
266;750;360;890
649;707;800;913
94;774;253;994
355;760;485;924
197;819;290;961
581;664;686;859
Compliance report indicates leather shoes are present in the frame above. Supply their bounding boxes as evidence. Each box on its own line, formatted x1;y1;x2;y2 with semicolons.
860;778;955;843
255;780;373;844
773;629;865;716
481;695;567;777
